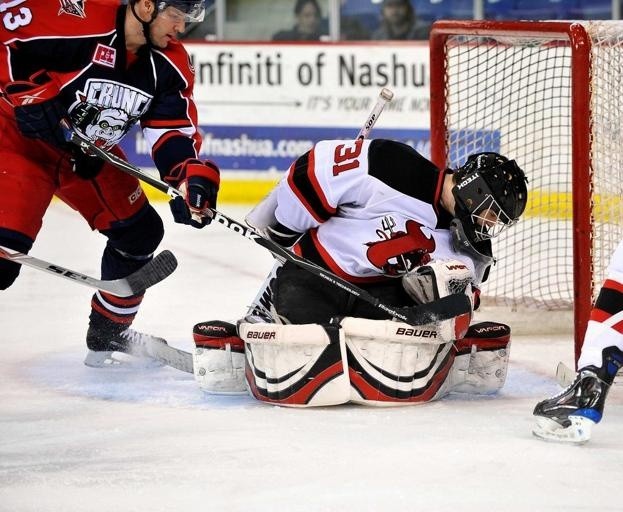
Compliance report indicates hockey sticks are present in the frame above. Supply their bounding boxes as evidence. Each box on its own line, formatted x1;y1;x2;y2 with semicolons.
69;131;471;326
1;244;177;298
141;89;392;375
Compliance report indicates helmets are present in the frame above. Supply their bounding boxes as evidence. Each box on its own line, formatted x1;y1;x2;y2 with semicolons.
452;152;527;244
151;0;205;24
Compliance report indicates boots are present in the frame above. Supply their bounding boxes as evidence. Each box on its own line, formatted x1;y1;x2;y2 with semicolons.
533;346;622;426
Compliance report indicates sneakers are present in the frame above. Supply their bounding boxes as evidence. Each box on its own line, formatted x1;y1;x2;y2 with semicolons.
87;325;167;354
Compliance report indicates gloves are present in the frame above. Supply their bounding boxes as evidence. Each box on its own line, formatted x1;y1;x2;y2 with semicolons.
4;69;68;141
167;158;220;229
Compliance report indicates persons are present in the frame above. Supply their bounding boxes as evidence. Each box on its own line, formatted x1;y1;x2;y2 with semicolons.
273;0;326;42
235;138;528;409
533;236;623;442
370;0;433;40
0;0;220;372
